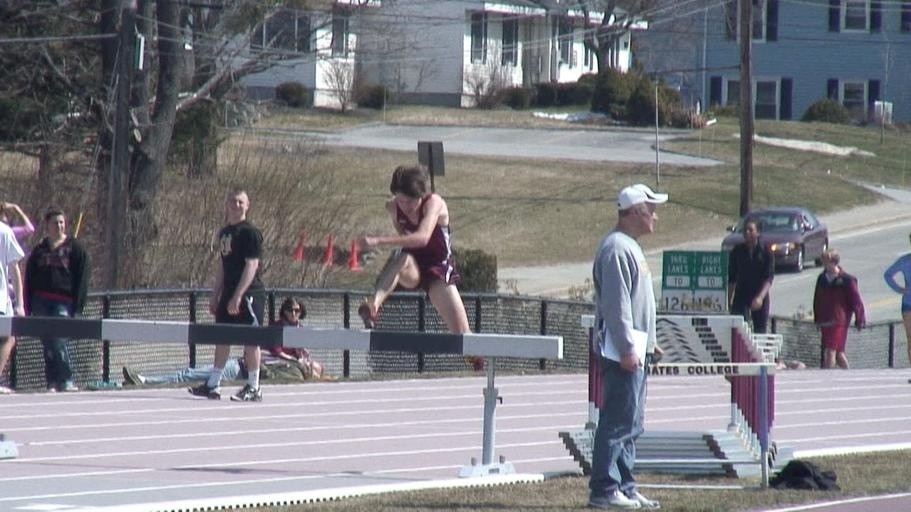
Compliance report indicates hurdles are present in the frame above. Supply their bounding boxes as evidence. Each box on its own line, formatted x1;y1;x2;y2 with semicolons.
0;315;565;479
557;313;795;477
631;361;775;491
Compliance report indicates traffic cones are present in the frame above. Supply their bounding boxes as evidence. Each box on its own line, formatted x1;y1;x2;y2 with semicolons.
294;232;307;256
346;237;357;267
321;234;334;264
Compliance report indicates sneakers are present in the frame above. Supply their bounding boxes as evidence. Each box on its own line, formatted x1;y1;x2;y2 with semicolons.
123;366;142;387
591;490;661;511
464;356;483;371
0;385;13;393
50;380;79;391
188;379;222;400
231;383;263;402
358;302;378;328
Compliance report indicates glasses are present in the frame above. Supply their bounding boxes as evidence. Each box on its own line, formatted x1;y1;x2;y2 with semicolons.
287;307;300;313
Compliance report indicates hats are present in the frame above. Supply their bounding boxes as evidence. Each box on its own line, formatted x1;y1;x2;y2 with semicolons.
617;183;668;212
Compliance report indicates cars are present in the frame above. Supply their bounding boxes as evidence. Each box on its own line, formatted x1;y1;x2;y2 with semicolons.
721;207;830;270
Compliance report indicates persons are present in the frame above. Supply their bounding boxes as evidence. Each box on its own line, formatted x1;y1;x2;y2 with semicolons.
123;350;271;381
883;233;911;384
1;200;91;393
268;298;323;381
588;185;670;509
188;191;264;402
728;219;776;334
814;249;866;368
787;216;798;231
359;168;487;372
